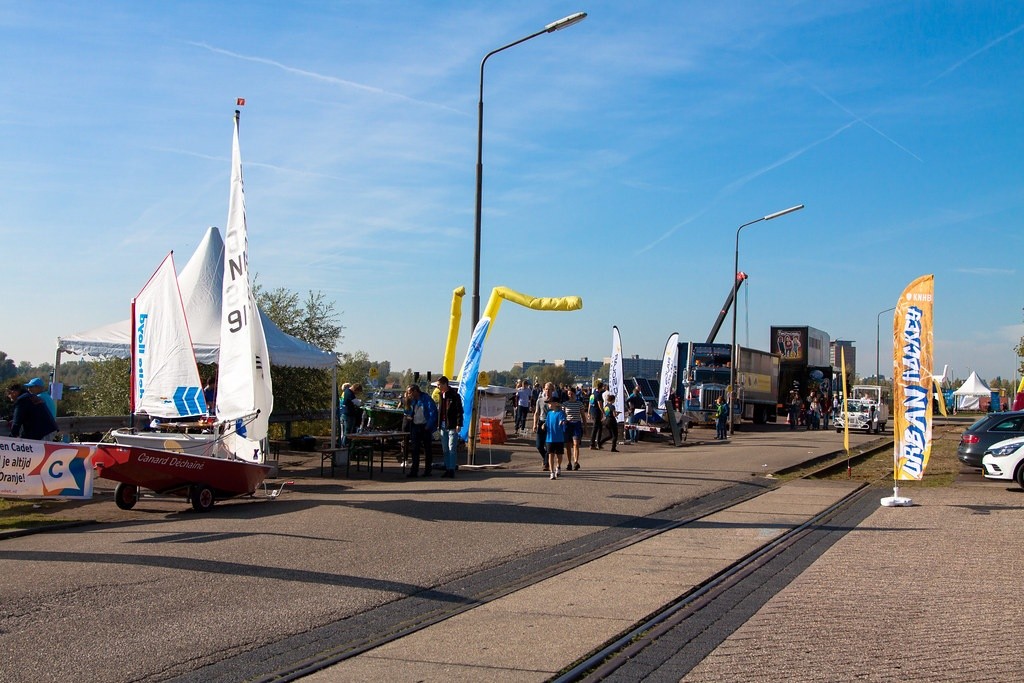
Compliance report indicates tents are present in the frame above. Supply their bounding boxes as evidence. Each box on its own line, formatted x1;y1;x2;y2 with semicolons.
52;227;337;447
953;371;991;408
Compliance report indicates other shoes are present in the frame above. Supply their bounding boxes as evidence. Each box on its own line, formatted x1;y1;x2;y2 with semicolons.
566;465;572;470
550;473;555;480
33;502;50;509
611;449;619;453
441;469;455;478
422;469;432;477
573;463;581;471
555;466;561;478
520;430;526;433
513;430;518;434
597;444;603;450
591;446;599;450
406;471;418;479
542;464;549;471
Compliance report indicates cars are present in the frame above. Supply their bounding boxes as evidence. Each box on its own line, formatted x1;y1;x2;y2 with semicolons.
981;435;1024;492
956;409;1024;467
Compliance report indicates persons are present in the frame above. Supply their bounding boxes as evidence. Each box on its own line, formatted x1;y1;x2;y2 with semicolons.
624;385;646;443
339;383;362;448
787;391;838;431
513;379;620;480
402;376;464;479
986;401;992;413
713;396;730;440
7;377;58;441
1003;402;1008;412
849;392;857;402
204;374;217;414
861;393;870;403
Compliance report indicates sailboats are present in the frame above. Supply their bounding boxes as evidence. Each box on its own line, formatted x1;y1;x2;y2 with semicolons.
91;103;275;512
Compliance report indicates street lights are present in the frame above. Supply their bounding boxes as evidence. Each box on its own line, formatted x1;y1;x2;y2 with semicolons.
472;12;589;341
728;204;806;434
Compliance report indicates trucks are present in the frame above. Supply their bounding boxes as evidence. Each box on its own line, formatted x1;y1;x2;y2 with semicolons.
833;385;890;435
681;343;781;431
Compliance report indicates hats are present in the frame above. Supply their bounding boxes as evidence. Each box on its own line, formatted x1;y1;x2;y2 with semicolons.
545;397;561;403
23;377;45;388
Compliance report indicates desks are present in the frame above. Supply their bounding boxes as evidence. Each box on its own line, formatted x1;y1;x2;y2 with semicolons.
344;430;410;473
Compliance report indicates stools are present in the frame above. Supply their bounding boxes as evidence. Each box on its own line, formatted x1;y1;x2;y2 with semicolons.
266;440;291;460
318;444;375;477
